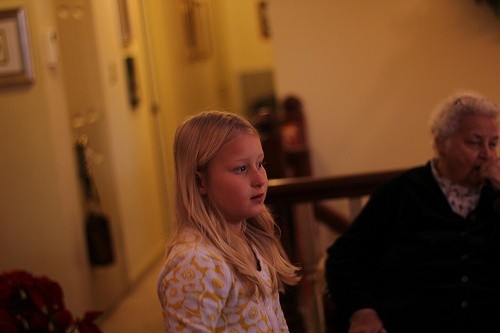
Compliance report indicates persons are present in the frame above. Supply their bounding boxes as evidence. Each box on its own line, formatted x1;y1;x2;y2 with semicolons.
324;92;500;333
156;108;300;333
